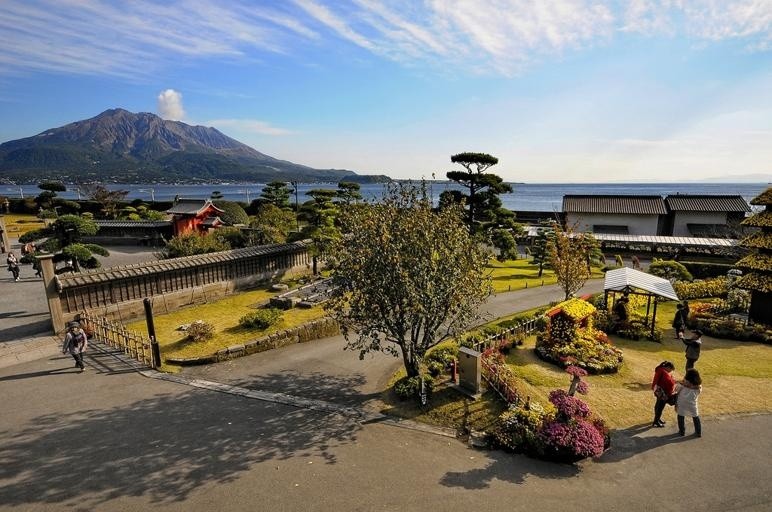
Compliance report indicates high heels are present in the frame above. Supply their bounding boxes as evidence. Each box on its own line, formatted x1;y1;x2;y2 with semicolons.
652;419;665;427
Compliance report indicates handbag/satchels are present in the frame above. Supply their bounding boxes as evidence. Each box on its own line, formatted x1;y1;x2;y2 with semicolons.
653;384;668;401
666;393;678;406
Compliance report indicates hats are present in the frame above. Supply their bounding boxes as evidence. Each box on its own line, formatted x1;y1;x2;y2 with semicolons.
691;330;703;337
70;321;80;329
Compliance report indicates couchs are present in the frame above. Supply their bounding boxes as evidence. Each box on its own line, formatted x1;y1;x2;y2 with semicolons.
269;277;341;309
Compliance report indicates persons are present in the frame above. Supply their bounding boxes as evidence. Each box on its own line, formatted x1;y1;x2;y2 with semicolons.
678;328;703;374
674;368;702;437
671;303;686;340
31;263;43;279
7;251;21;281
683;301;689;314
62;321;88;371
650;360;677;427
